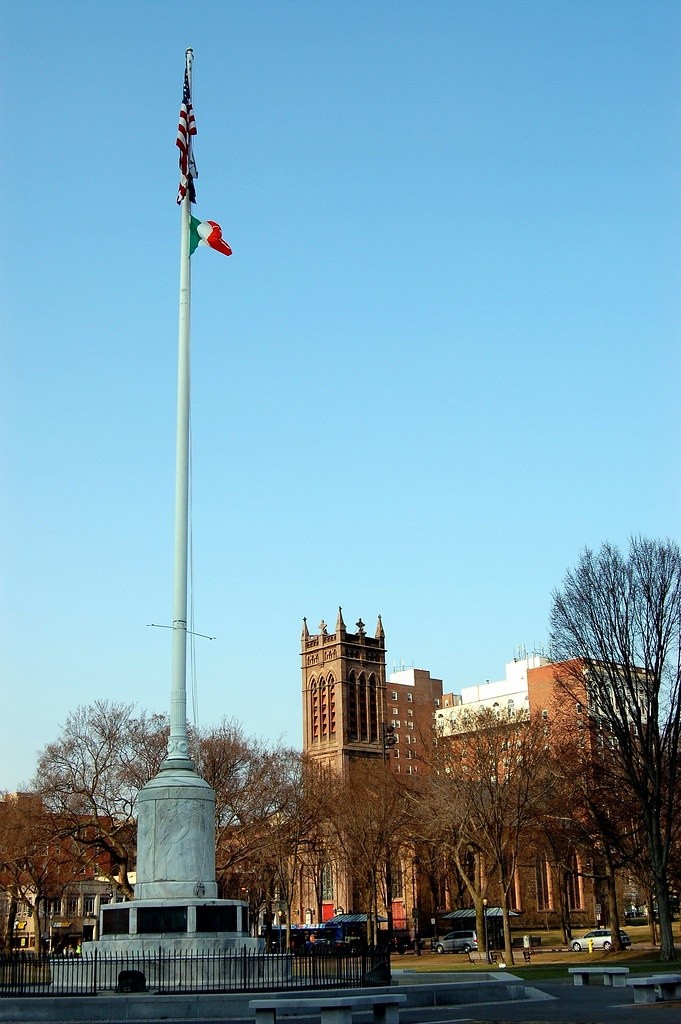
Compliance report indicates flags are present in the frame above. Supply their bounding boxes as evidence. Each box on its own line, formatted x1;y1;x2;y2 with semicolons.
175;62;200;206
190;214;232;260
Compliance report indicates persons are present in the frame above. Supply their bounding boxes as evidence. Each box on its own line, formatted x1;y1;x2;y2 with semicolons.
57;941;81;959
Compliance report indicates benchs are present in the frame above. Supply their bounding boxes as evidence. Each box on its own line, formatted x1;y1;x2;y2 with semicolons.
568;967;629;987
626;974;681;1003
247;994;407;1024
467;949;531;964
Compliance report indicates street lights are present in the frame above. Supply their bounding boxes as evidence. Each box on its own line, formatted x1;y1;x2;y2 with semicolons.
380;722;400;931
483;899;492;964
277;908;284;956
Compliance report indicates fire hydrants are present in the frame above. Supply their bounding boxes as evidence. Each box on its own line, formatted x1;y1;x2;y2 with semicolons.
588;939;594;954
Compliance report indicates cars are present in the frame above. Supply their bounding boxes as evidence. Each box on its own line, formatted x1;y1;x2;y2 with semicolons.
266;933;407;957
435;930;485;954
624;909;644;917
570;929;631;953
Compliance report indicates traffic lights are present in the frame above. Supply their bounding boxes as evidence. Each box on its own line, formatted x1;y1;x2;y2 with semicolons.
28;908;32;918
